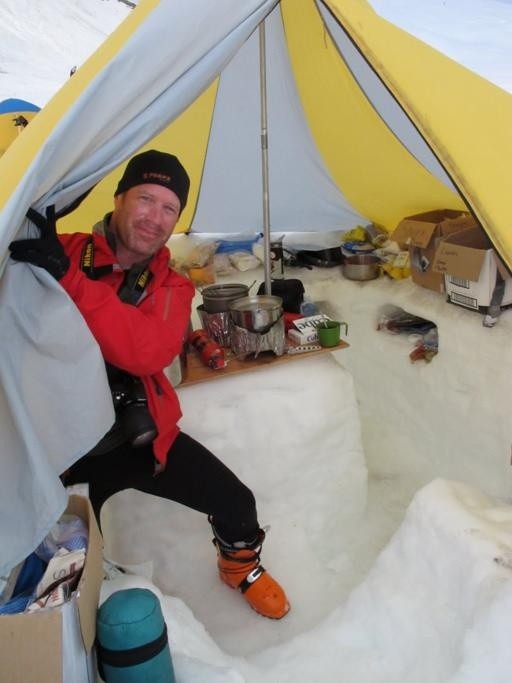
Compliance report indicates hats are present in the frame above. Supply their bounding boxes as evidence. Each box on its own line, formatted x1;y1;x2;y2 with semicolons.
112;149;190;213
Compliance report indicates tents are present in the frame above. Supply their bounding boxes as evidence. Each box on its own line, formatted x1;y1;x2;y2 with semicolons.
4;0;512;683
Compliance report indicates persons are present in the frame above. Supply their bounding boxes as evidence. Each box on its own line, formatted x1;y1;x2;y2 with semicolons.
6;146;295;620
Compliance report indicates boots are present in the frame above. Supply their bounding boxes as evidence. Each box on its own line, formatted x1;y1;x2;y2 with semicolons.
216;527;290;623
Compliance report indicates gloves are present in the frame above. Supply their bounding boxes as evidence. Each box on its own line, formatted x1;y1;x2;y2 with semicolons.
8;203;69;281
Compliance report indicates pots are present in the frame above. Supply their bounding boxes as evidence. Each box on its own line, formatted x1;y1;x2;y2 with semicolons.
192;278;283;362
342;255;382;280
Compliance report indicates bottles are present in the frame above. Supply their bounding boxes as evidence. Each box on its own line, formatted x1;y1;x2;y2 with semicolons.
190;328;228;371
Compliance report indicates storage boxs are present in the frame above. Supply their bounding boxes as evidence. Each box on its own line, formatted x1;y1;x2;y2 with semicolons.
430;224;512;313
0;494;105;683
388;208;475;292
188;263;216;288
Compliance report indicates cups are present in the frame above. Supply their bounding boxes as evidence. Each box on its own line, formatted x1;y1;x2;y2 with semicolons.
315;321;349;348
